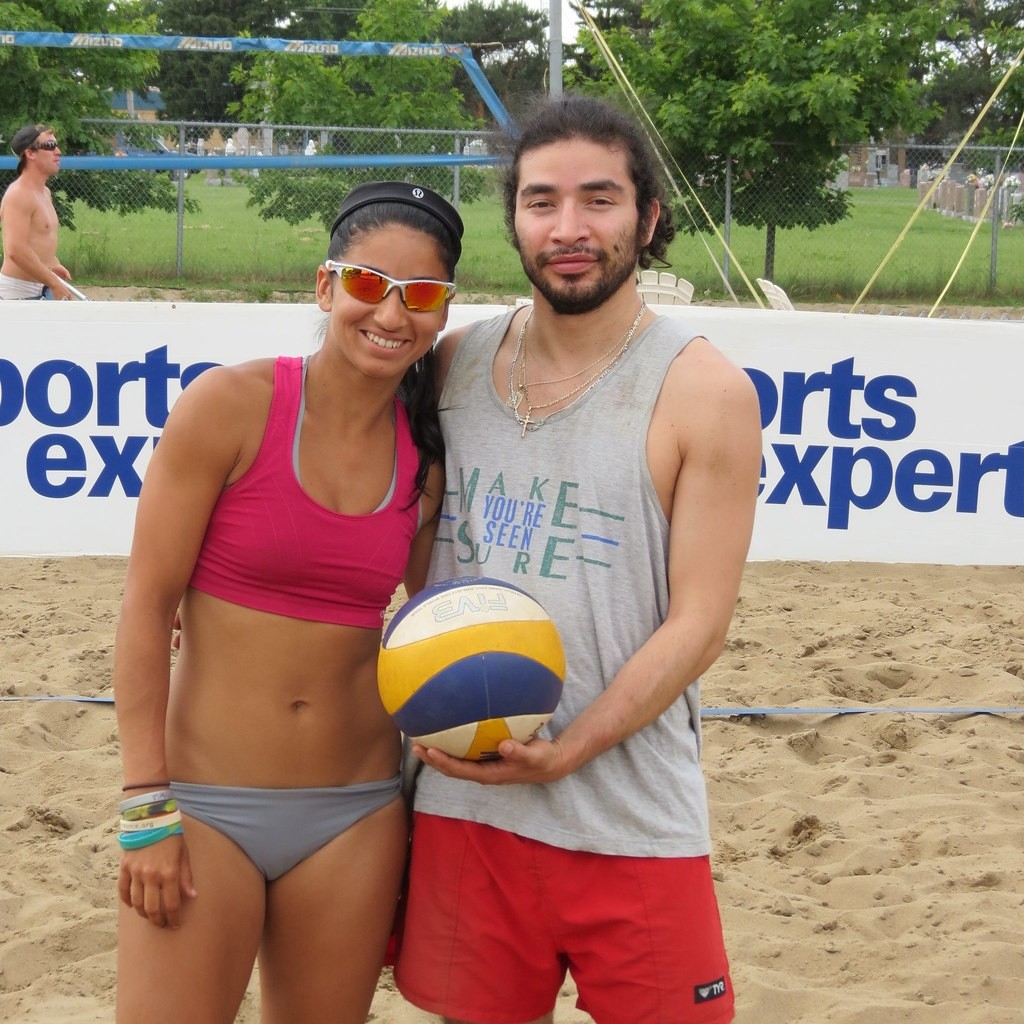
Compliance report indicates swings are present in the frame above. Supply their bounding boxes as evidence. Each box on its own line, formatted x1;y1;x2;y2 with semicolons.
574;0;1024;320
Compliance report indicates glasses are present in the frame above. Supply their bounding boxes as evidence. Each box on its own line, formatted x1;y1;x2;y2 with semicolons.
326;260;456;313
31;141;59;151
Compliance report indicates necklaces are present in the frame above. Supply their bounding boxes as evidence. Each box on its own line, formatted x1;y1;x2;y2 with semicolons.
507;300;647;438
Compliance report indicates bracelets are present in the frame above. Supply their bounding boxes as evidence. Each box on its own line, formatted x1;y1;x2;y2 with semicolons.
117;780;184;850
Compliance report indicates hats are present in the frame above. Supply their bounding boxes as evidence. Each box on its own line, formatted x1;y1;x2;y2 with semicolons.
11;124;48;174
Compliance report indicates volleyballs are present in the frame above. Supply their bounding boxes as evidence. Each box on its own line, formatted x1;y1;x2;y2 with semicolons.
376;573;569;763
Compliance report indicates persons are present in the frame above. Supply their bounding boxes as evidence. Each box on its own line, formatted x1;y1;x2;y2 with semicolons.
173;96;765;1024
116;181;464;1024
0;123;72;300
